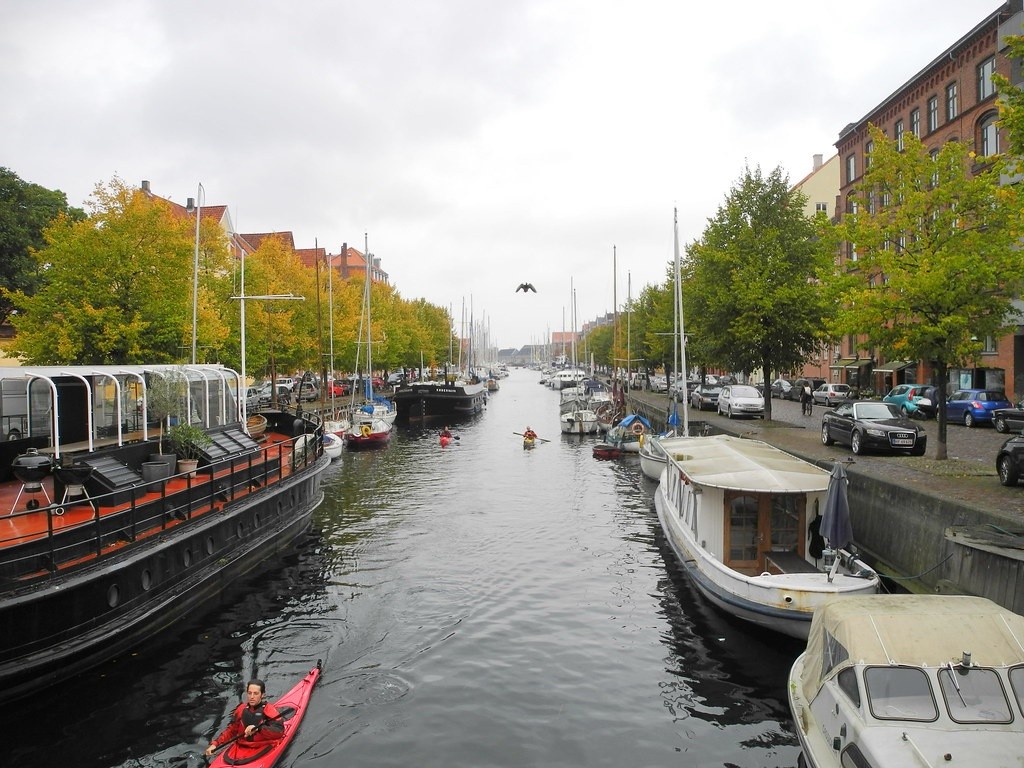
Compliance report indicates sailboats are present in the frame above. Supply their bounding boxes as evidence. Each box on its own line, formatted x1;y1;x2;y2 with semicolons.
539;246;656;451
301;232;399;460
446;290;510;392
640;208;690;480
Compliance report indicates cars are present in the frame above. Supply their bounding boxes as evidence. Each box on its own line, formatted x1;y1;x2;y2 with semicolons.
235;367;444;413
821;399;928;456
668;380;765;421
812;383;850;407
882;384;934;417
936;389;1012;427
599;365;738;394
770;378;797;400
995;429;1024;488
991;400;1024;434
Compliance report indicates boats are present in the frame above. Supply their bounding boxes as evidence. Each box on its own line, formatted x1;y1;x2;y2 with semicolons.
789;593;1024;768
208;657;323;768
440;436;452;448
656;434;882;642
523;436;536;451
0;366;329;709
593;445;624;461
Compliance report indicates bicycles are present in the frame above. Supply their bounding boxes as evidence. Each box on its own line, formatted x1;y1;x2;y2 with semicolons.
799;394;812;416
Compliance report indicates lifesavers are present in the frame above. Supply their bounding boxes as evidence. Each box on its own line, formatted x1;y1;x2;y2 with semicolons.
632;422;644;435
640;435;644;448
361;426;371;437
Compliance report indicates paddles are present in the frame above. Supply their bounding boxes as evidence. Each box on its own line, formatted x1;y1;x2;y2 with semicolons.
200;706;296;758
423;429;461;440
513;431;552;442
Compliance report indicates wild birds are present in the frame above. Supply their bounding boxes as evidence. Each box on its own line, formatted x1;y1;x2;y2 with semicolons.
515;282;536;294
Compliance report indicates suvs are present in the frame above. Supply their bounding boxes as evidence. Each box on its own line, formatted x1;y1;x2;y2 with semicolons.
789;377;826;402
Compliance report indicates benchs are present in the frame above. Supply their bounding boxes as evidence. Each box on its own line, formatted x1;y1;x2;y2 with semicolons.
762;550;823;575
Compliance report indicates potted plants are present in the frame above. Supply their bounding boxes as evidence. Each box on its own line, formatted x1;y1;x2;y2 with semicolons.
141;358;194;476
162;418;216;478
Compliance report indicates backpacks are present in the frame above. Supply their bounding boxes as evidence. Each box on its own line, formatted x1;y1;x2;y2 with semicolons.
804;386;811;396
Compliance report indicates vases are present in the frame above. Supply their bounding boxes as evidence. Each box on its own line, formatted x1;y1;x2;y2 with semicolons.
142;461;170;492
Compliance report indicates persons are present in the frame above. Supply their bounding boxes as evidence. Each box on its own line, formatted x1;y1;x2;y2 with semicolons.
799;382;814;413
523;426;538;439
438;426;453;438
206;679;285;756
